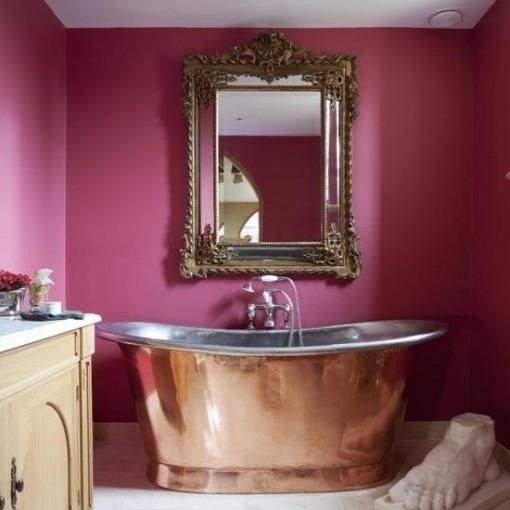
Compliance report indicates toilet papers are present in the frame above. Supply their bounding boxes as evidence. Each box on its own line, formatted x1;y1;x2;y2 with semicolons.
41;301;62;316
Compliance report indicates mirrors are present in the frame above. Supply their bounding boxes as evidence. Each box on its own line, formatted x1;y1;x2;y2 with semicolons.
177;27;361;284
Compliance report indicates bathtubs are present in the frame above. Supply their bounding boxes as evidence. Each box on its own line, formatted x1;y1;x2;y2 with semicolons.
95;318;449;493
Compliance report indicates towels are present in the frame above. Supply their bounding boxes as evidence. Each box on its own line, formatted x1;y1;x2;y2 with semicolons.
18;309;83;322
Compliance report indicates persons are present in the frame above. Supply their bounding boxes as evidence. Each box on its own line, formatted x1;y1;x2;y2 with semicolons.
385;412;500;510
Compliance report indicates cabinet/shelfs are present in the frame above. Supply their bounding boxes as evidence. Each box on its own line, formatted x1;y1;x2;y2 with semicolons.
1;321;98;510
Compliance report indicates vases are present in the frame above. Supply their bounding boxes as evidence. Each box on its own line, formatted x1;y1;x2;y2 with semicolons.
0;287;27;316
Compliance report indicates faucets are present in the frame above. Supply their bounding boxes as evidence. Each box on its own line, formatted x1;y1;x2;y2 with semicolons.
248;300;291;327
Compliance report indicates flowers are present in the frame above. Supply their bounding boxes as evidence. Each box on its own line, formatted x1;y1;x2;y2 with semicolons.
0;270;31;293
